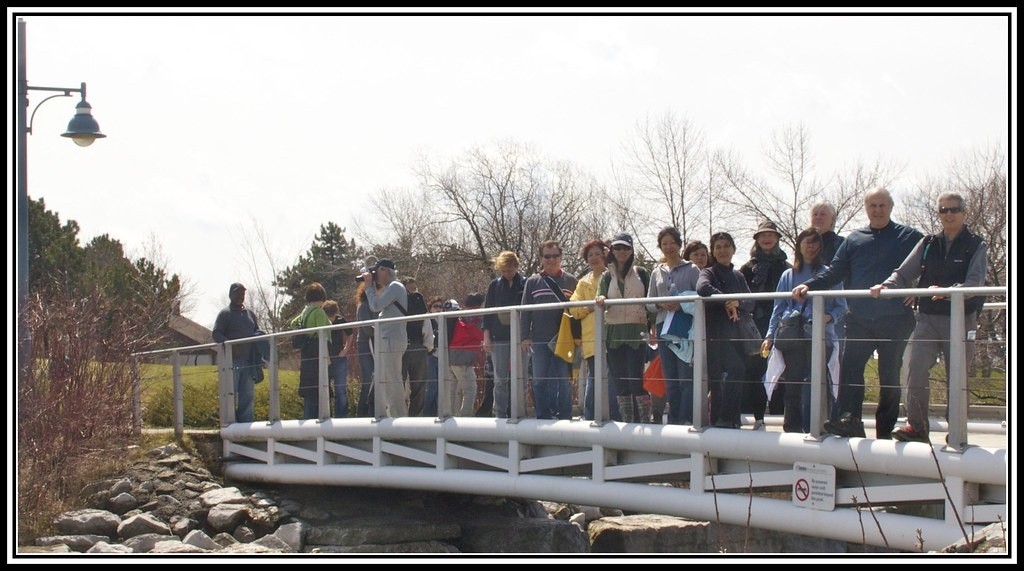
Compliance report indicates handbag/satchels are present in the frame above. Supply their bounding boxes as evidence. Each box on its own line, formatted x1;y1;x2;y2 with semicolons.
498;312;510;326
737;312;763;355
775;317;805;348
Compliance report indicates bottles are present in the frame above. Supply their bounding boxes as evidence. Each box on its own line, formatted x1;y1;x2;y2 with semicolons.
640;332;659;351
781;310;791;320
789;310;800;317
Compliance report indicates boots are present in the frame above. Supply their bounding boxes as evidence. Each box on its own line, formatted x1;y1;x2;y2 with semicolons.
617;393;634;423
636;395;652;422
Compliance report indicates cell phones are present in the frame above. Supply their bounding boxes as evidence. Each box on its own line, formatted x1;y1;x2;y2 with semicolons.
526;345;534;357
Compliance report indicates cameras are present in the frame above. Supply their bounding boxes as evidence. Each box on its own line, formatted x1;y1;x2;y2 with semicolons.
355;265;376;282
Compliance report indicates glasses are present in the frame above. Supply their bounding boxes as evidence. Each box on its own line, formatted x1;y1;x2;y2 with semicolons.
613;245;630;250
432;304;443;309
939;207;961;214
543;254;559;258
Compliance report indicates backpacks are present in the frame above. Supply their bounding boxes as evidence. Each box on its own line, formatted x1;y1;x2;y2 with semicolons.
292;307;320;348
395;287;428;340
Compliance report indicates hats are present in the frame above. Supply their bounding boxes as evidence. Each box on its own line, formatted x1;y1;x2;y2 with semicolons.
230;283;246;293
368;259;394;269
445;300;458;308
753;222;782;239
611;233;632;247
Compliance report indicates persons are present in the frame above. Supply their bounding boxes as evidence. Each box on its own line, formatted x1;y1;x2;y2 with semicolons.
212;283;260;422
742;222;793;416
760;228;849;433
401;276;494;417
519;239;579;419
481;250;528;418
594;233;656;423
648;232;768;430
568;240;623;422
792;187;925;439
289;282;333;419
355;280;385;418
321;301;354;418
870;193;989;443
362;259;409;418
646;226;701;424
809;200;848;418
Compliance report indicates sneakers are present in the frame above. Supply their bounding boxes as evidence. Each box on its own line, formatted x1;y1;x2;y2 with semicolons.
891;426;928;443
825;415;866;437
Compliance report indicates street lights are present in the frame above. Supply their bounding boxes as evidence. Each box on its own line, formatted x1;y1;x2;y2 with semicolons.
18;19;108;402
